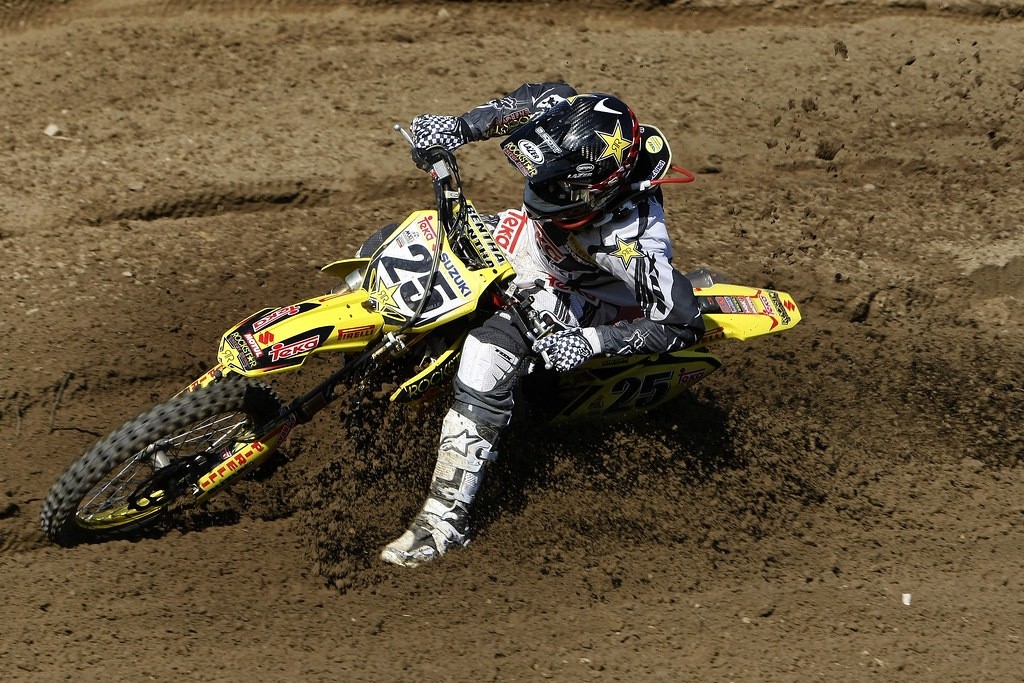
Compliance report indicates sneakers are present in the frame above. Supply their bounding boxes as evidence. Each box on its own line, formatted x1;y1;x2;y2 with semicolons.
378;493;473;568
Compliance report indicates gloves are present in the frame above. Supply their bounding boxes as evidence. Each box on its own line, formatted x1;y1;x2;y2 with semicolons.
409;113;466;152
531;327;592;372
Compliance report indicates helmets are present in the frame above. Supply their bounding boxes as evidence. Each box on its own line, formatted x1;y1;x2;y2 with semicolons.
499;93;641;230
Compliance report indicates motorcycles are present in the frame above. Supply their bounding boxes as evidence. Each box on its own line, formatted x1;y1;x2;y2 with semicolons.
39;110;803;550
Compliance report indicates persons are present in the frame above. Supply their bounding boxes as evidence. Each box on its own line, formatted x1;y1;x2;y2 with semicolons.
298;82;706;569
